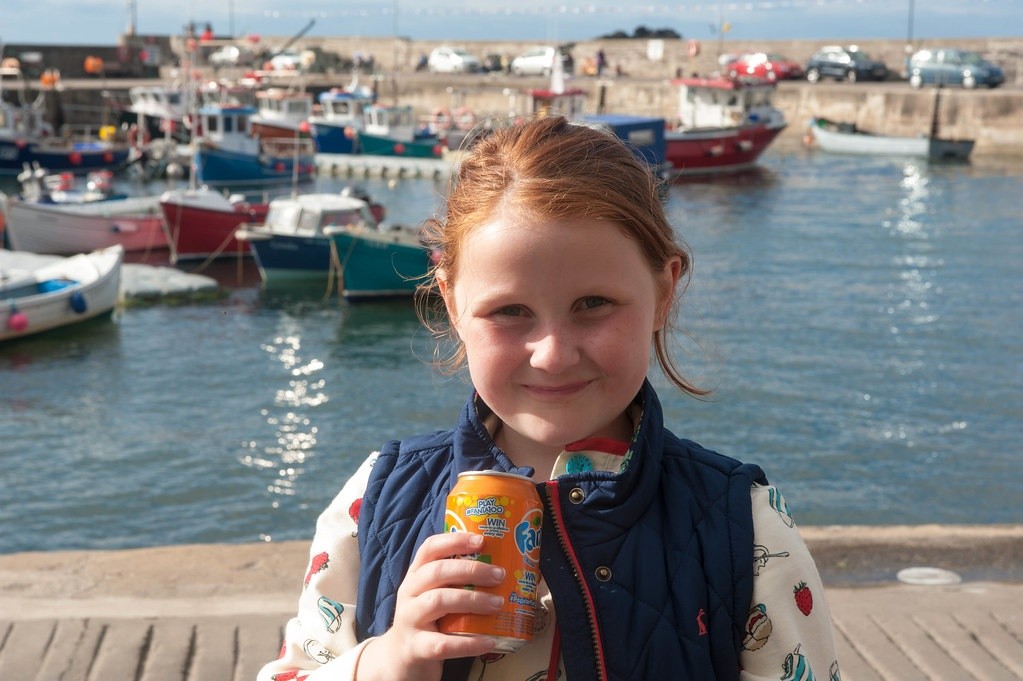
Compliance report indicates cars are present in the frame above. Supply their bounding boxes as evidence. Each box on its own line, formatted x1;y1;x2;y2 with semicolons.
511;41;576;75
902;47;1005;88
804;45;888;83
428;47;482;74
723;50;804;83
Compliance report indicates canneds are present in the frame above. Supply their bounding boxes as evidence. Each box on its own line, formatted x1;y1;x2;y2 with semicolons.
437;469;543;654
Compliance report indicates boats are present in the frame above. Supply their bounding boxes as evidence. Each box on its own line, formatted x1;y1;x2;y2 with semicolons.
0;77;666;255
236;193;379;290
158;181;385;266
323;222;441;304
0;247;123;343
803;72;975;164
502;47;789;178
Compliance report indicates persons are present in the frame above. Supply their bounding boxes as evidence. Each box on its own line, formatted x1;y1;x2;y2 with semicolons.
256;114;846;681
